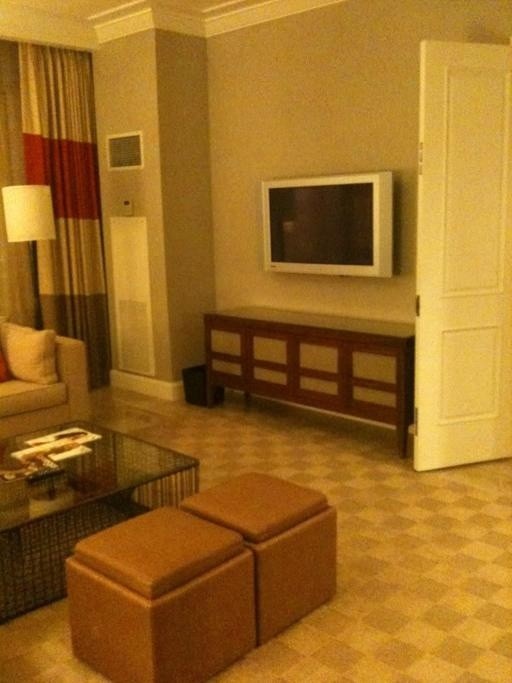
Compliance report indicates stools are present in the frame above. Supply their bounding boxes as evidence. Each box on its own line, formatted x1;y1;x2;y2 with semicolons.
179;472;339;646
64;504;254;681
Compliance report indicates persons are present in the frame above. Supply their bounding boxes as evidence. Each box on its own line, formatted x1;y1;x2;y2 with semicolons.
28;431;87;461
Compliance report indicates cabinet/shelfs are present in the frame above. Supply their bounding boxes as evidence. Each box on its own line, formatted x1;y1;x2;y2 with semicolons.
204;304;415;458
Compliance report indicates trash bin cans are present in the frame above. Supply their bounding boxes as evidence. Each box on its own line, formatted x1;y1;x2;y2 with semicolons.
183;365;224;405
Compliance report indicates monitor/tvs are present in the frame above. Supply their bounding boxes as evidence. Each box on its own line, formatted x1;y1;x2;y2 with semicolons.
261;171;393;278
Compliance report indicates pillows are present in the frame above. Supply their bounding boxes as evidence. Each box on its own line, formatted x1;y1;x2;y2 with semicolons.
6;324;59;383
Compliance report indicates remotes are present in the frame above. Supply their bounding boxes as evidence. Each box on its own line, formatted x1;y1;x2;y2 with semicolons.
25;467;65;482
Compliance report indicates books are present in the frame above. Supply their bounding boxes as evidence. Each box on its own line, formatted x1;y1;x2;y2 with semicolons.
23;452;65;483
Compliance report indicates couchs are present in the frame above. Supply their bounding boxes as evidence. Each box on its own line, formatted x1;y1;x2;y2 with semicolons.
0;311;89;439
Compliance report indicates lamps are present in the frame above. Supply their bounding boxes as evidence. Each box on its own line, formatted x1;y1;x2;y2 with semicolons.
0;184;62;326
